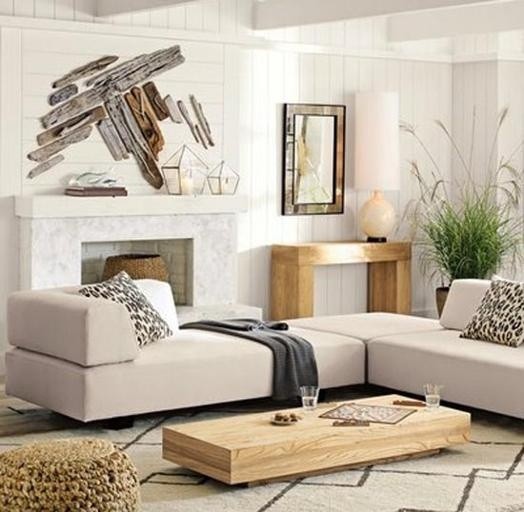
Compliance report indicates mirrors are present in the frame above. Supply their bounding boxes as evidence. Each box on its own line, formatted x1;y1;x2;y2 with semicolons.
281;101;347;217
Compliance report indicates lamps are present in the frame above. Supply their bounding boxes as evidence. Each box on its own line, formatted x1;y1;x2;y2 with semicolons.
358;189;398;244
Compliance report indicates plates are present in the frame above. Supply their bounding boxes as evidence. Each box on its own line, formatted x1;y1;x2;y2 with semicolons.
270;417;303;425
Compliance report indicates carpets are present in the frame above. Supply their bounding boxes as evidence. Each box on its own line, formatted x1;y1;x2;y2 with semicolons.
0;410;524;512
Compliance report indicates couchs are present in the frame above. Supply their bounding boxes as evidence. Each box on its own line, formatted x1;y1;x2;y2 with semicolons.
4;277;524;429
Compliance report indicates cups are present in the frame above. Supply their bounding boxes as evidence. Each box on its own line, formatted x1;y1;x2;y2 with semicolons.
299;385;320;413
425;383;440;411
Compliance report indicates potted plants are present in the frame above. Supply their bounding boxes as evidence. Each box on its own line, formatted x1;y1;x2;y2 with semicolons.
401;106;522;321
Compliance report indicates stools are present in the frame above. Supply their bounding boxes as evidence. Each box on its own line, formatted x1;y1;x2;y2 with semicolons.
0;439;142;511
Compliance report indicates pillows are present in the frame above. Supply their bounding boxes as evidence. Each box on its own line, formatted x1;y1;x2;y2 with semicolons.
459;277;524;346
77;274;174;350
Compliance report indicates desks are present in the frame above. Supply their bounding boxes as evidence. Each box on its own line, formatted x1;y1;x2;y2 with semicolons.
268;240;412;319
